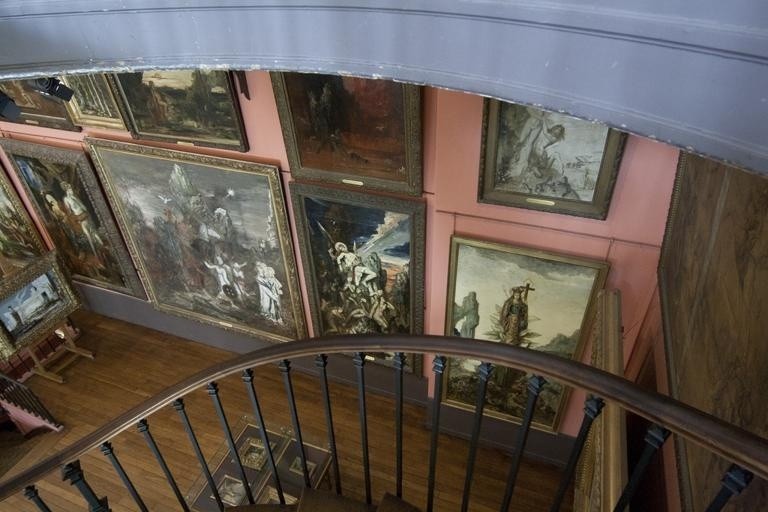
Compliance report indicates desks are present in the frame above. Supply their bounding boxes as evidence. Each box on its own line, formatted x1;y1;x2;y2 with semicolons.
188;414;334;512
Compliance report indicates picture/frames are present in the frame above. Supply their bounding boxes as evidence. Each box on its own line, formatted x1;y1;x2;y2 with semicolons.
255;485;299;505
0;251;83;363
440;234;611;436
104;70;250;153
84;135;309;345
0;168;48;276
230;437;277;471
210;474;252;507
0;136;152;303
476;96;629;221
0;75;82;132
656;148;768;512
269;72;423;198
57;74;128;132
287;180;426;378
289;456;318;479
